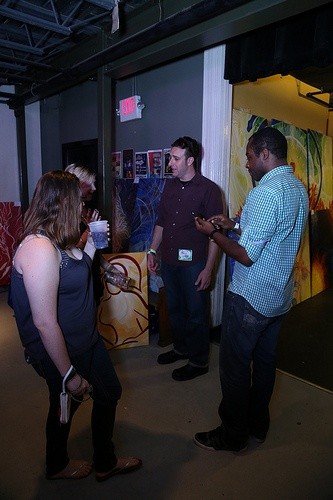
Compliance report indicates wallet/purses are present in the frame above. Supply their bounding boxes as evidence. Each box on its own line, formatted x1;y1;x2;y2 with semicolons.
58;392;69;423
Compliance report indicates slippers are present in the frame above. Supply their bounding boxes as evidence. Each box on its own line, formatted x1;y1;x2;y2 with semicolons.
44;461;92;480
94;456;141;483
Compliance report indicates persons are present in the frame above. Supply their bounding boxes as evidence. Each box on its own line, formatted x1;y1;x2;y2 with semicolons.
8;169;143;483
147;135;224;383
63;162;125;310
192;126;311;452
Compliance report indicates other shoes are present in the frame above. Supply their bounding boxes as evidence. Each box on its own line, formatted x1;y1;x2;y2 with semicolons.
157;350;188;364
193;430;246;452
242;419;265;442
171;363;208;381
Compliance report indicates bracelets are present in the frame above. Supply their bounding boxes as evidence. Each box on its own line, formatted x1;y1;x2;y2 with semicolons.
145;247;157;255
233;222;240;233
59;365;77;384
207;228;219;240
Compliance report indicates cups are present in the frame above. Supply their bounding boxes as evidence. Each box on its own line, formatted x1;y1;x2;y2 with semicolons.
88;221;108;249
